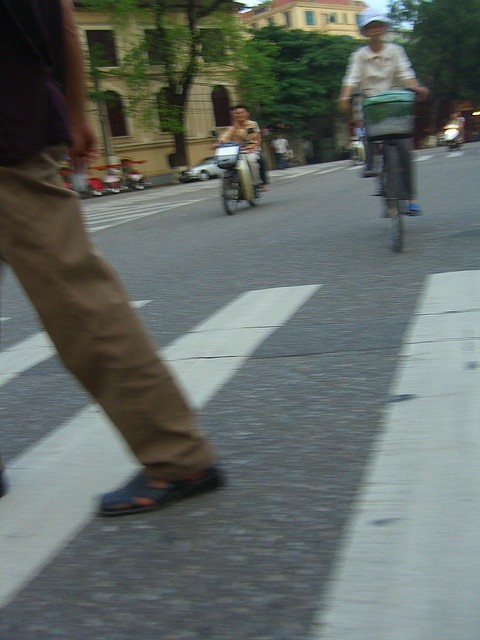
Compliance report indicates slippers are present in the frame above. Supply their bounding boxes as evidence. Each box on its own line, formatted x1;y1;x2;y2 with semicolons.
405;204;420;216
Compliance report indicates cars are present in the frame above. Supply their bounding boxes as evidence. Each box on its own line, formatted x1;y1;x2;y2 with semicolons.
175;155;226;182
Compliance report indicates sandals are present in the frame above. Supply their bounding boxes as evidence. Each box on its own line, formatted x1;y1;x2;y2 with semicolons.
97;469;222;515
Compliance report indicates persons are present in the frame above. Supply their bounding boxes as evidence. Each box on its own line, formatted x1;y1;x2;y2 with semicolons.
211;103;270;192
0;0;224;518
357;121;366;143
271;133;290;171
445;111;466;144
336;5;429;217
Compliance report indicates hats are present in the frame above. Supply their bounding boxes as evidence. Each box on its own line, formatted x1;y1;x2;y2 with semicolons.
358;6;392;32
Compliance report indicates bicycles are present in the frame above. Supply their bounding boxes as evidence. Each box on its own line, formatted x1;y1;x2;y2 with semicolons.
347;87;420;255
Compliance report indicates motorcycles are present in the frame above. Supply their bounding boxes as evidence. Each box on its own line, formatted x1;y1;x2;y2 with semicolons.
349;136;367;167
205;128;262;215
443;120;464;149
